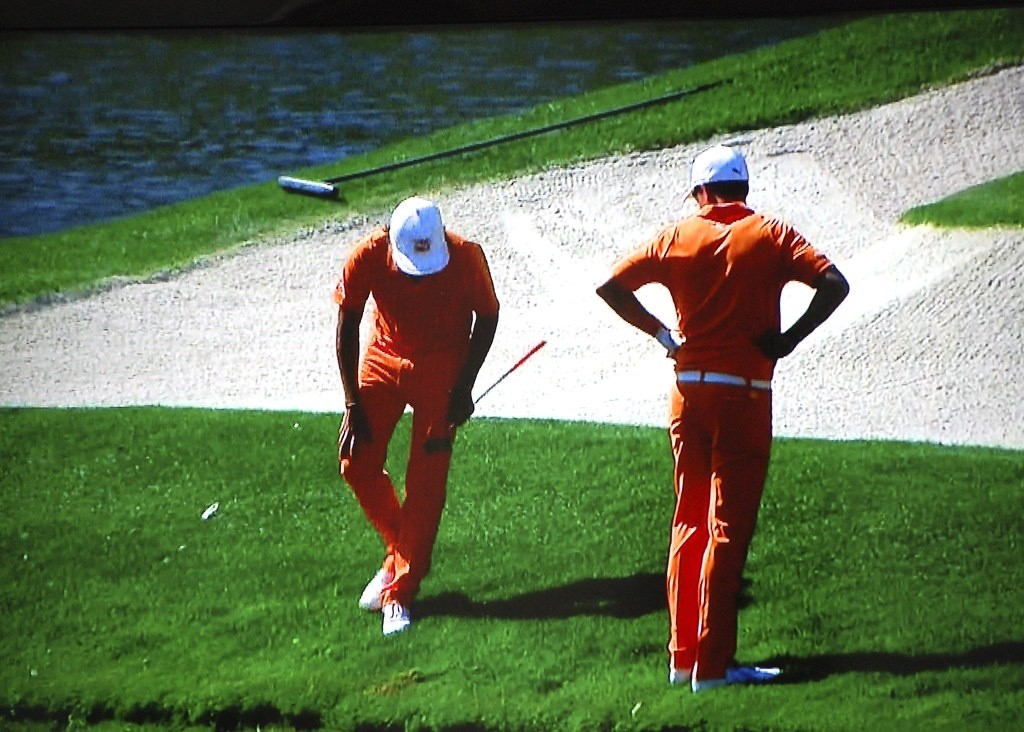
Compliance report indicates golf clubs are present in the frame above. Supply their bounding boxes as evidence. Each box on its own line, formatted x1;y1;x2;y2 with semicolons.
419;340;548;457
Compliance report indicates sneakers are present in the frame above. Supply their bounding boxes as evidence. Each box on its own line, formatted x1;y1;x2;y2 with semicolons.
359;567;393;612
690;661;780;694
383;599;410;637
668;665;692;687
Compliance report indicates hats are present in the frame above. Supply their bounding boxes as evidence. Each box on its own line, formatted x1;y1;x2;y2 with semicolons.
682;145;749;202
390;197;449;276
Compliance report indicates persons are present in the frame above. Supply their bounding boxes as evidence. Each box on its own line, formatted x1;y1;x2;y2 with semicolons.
332;196;499;634
595;144;849;692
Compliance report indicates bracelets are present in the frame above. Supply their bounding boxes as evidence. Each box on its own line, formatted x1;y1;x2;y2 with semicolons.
346;402;356;409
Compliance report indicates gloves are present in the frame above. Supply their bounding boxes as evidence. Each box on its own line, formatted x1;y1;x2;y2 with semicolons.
655;326;680;359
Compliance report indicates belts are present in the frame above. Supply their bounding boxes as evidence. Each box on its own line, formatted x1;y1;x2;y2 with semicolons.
677;370;771;390
